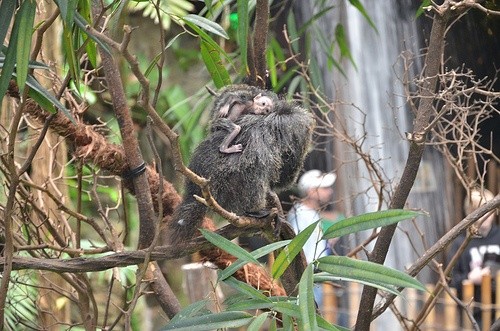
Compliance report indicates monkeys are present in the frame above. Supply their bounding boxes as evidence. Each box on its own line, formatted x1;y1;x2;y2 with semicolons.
164;96;316;256
208;83;278;154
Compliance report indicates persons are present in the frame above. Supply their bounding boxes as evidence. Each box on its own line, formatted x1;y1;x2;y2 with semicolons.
444;185;500;331
287;169;337;318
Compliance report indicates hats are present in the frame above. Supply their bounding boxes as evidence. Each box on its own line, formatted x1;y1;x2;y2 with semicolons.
298;170;337;191
